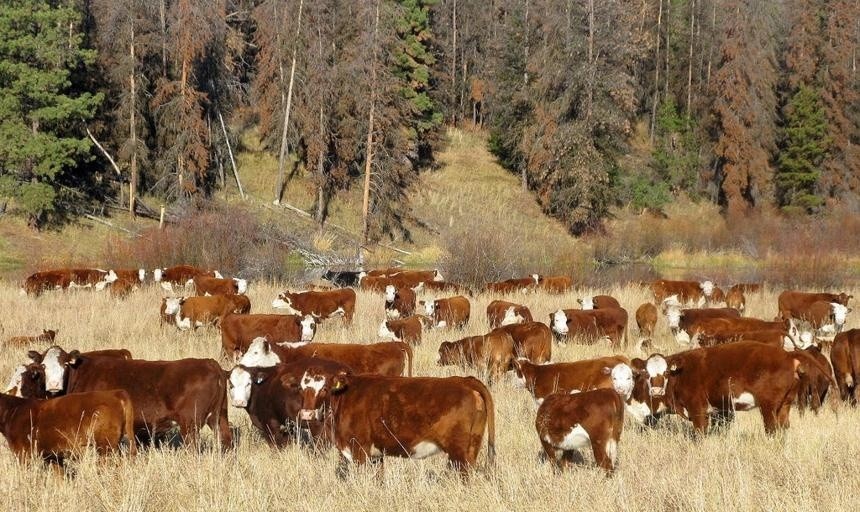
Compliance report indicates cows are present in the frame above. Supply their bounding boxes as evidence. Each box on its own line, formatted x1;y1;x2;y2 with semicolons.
0;264;860;481
